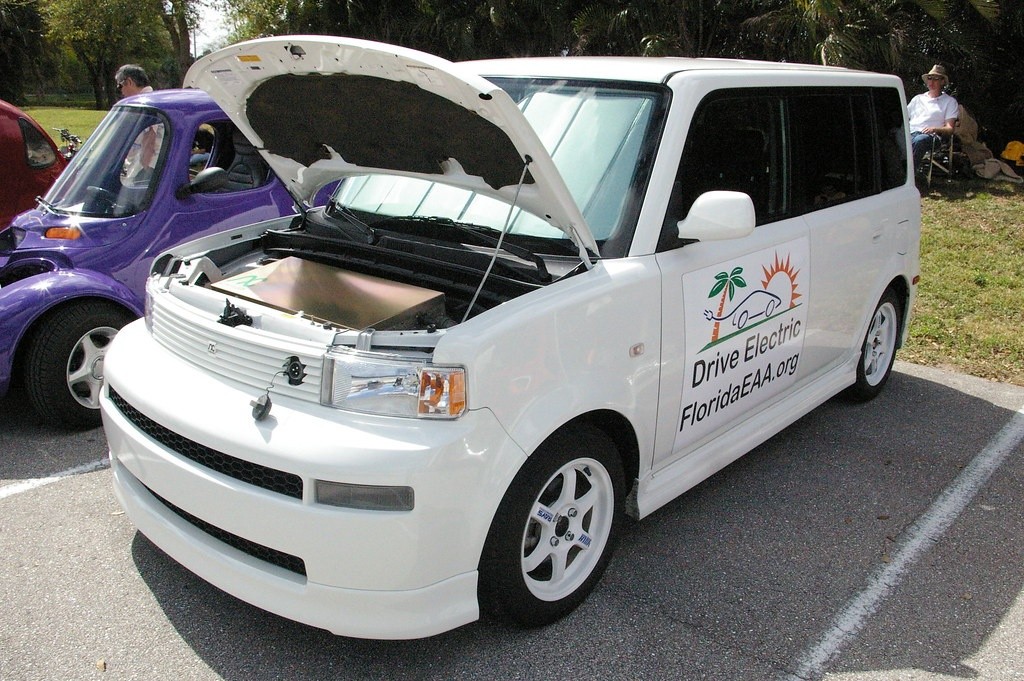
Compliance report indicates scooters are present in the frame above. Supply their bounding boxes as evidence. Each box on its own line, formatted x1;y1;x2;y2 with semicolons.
50;127;82;161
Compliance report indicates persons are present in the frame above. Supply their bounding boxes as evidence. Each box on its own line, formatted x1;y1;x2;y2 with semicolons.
188;123;215;181
907;64;959;173
113;64;164;217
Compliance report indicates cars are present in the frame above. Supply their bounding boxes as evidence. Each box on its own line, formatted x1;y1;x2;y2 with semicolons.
0;88;342;433
0;98;69;234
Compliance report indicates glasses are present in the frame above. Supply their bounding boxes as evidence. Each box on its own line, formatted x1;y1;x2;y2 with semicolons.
927;75;941;81
117;79;136;90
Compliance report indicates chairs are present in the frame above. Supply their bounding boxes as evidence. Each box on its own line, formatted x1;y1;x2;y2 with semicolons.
215;130;265;194
917;104;964;189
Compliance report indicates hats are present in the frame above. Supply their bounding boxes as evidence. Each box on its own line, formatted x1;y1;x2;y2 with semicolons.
921;64;948;86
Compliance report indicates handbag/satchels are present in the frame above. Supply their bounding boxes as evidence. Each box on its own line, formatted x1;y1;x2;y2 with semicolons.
1001;141;1024;166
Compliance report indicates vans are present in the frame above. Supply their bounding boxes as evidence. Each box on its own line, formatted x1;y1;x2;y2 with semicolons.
99;35;921;643
